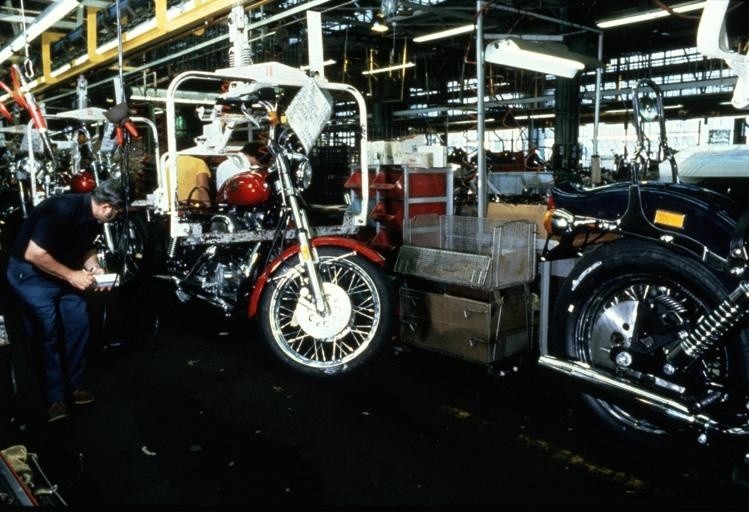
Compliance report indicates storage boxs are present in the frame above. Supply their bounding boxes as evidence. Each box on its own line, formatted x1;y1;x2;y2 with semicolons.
394;212;538;364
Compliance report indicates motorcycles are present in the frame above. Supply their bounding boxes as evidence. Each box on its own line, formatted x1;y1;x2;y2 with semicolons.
448;145;492;215
539;181;749;445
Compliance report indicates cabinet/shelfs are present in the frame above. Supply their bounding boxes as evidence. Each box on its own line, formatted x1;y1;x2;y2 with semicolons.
345;165;456;331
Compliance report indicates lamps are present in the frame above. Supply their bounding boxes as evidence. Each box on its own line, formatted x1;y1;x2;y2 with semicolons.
298;24;481;79
482;35;588;84
593;0;718;30
0;1;199;112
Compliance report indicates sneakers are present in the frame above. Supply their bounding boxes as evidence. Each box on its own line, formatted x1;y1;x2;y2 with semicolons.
43;382;98;426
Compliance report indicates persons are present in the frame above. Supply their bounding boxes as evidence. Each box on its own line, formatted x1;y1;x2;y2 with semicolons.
211;139;270;191
165;136;211;216
0;177;128;422
